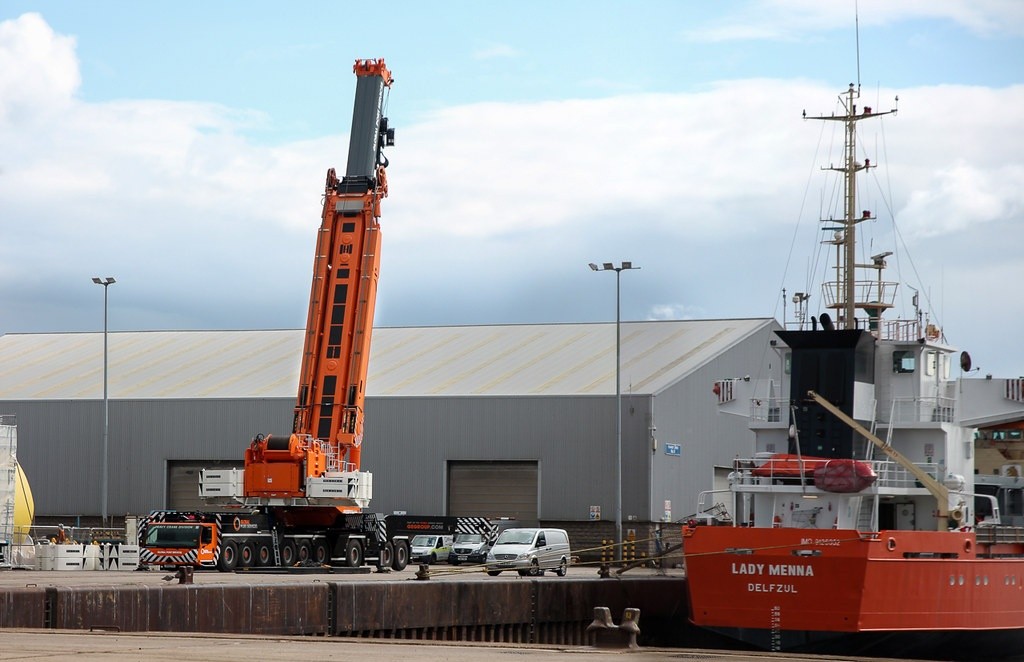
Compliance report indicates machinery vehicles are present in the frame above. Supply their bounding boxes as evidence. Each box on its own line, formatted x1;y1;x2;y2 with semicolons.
133;56;412;572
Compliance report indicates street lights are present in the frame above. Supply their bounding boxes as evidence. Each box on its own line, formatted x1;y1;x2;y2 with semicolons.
589;262;635;569
90;275;118;537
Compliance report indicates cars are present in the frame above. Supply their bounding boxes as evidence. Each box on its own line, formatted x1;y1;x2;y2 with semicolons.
410;534;455;566
447;533;489;566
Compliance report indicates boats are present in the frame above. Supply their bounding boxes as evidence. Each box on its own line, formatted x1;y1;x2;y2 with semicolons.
812;459;879;493
675;7;1024;661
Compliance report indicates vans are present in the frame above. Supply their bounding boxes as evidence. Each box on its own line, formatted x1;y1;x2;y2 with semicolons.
484;527;572;576
